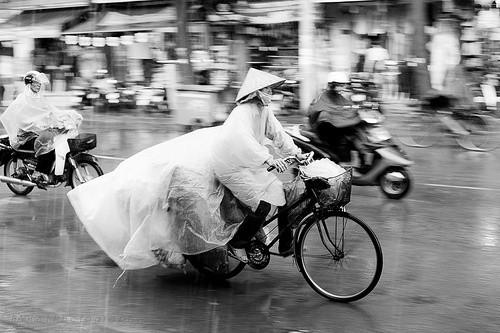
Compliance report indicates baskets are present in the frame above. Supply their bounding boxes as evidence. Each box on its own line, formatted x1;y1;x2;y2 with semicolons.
301;165;353;209
67;133;96;152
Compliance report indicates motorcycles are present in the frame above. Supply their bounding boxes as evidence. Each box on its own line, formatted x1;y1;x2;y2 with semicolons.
0;122;104;195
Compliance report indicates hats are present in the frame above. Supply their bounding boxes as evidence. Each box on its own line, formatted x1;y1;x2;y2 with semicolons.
235;67;287;103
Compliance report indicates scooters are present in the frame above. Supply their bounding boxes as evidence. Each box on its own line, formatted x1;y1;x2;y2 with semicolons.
284;94;414;202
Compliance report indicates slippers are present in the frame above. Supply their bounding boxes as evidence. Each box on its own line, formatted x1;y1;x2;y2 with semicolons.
31;175;48;184
229;244;250;264
282;245;307;257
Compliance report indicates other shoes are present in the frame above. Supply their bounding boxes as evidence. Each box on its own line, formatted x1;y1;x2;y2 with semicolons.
350;167;364;177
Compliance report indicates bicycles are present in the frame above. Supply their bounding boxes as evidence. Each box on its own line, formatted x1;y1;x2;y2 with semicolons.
397;107;500;152
192;150;384;302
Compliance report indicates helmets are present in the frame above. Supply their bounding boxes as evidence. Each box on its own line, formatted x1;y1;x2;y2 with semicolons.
325;70;353;85
24;71;41;84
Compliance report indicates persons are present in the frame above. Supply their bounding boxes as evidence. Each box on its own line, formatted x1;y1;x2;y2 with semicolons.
163;37;178;60
211;67;310;263
16;70;74;182
423;12;477;135
308;72;374;178
363;39;389;73
